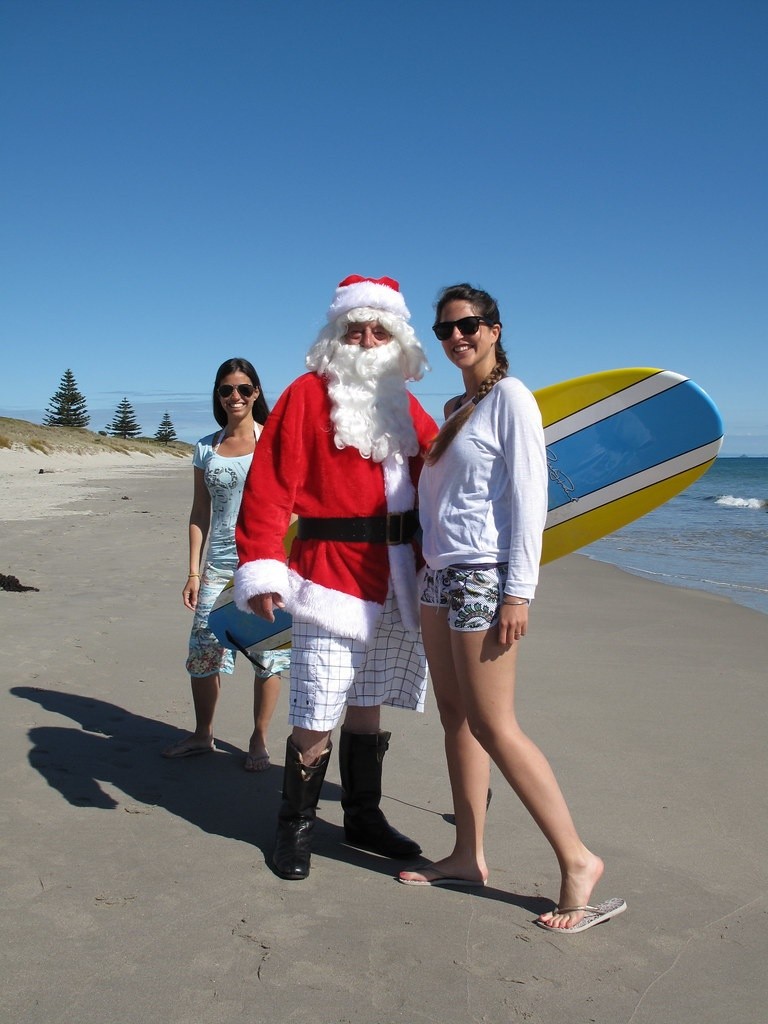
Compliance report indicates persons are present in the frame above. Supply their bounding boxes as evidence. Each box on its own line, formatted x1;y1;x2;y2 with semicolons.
159;358;299;771
398;283;627;933
233;275;441;880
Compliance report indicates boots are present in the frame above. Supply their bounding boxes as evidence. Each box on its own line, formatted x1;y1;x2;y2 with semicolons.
340;723;423;860
271;734;336;879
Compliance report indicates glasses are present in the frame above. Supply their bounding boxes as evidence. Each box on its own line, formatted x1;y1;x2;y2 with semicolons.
431;315;489;341
217;383;256;399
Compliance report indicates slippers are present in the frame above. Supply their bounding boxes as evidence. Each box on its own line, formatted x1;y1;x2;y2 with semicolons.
243;751;270;772
159;740;217;757
538;897;627;933
398;865;490;886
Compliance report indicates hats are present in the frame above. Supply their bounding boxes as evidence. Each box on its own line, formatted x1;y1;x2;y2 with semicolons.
326;275;412;322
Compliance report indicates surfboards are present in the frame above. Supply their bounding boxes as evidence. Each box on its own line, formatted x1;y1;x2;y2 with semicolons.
202;366;725;670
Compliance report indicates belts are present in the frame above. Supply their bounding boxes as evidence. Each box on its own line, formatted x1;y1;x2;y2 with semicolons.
296;510;421;547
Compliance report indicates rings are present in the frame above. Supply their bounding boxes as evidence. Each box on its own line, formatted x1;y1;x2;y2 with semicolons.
515;632;521;635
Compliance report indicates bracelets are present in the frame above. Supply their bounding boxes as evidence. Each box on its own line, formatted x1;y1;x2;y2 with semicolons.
188;574;200;577
502;598;528;605
